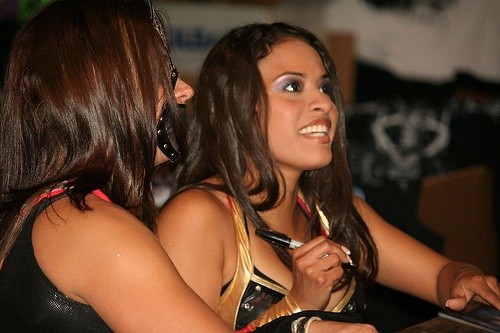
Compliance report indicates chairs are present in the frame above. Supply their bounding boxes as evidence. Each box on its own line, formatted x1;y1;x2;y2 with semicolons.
417;164;499;284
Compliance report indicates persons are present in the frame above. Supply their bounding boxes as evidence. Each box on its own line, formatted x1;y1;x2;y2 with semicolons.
1;1;379;333
151;22;499;333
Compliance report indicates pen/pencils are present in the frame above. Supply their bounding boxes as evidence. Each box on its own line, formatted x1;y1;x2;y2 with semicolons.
254;229;330;261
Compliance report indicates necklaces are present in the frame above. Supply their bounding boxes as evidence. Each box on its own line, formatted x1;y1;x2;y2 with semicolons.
243;189;332;274
